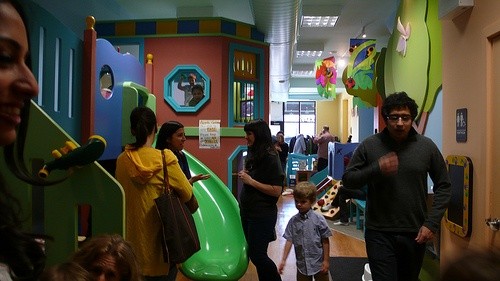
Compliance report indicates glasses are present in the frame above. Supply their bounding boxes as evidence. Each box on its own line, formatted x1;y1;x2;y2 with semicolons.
386;115;412;122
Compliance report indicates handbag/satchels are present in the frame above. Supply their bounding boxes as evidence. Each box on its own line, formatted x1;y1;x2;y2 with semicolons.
153;150;200;263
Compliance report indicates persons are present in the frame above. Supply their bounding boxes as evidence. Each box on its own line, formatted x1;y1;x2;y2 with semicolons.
178;73;204;105
321;185;367;225
272;125;306;172
188;84;205;106
313;125;334;172
342;91;451;281
0;0;210;281
278;181;333;281
238;119;285;281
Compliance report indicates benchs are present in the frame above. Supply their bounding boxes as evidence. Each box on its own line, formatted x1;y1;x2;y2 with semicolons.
349;197;366;231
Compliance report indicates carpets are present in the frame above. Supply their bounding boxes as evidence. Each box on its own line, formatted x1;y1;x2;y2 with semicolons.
328;255;370;281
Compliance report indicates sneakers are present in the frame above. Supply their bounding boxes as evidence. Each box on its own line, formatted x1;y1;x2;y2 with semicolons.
333;219;349;226
322;203;338;211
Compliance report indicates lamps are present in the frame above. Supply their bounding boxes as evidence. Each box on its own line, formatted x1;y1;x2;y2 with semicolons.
362;26;367;38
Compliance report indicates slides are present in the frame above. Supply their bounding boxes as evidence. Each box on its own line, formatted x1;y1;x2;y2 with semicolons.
139;137;249;281
